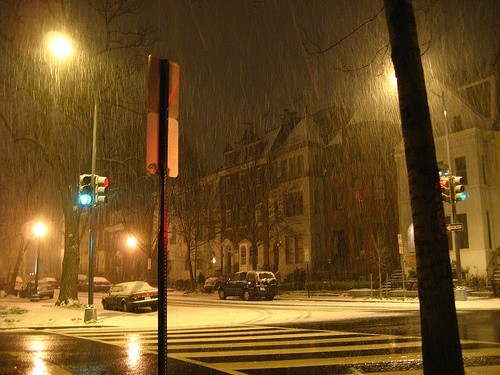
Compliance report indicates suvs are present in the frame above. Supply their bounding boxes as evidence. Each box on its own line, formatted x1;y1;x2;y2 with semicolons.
218;270;280;301
204;276;227;293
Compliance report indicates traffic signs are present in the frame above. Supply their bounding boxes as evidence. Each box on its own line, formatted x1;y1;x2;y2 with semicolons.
446;223;465;231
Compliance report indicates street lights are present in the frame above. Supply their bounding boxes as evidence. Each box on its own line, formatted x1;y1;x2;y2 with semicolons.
389;72;463;287
46;35;99;308
33;221;46;289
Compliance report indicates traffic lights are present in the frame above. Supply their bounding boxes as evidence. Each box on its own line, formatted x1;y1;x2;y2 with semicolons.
452;176;467;204
440;175;450;203
77;173;95;207
94;174;109;206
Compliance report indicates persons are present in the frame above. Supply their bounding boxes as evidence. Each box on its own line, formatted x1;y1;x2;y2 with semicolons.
198;272;205;292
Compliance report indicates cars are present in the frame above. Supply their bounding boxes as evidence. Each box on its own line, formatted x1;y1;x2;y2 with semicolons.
92;276;112;293
101;281;159;313
77;274;89;292
19;279;54;300
42;277;59;289
13;276;24;296
